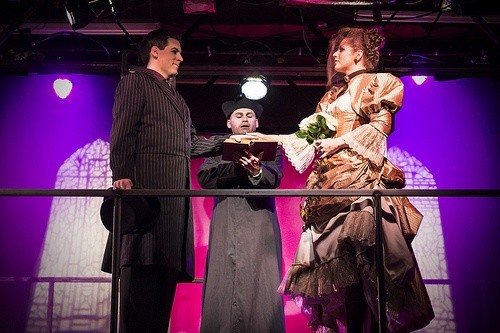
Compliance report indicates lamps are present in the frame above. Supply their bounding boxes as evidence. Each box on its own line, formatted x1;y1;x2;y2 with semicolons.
63;0;119;31
239;72;271;102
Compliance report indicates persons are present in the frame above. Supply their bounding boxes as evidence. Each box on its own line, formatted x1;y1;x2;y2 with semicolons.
247;28;435;333
198;98;285;333
101;30;262;333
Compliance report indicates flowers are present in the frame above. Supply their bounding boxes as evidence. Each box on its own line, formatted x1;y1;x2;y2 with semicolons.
295;112;338;166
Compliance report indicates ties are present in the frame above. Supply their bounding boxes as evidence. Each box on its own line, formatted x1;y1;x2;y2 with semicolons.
166;79;178;102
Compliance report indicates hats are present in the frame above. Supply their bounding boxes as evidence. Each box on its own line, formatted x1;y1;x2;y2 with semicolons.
99;184;162;236
221;97;264;120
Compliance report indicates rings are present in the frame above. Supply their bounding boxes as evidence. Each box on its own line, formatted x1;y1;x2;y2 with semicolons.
319;149;323;154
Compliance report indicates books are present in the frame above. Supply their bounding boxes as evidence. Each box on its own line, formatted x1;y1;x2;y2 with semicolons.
222;137;278;161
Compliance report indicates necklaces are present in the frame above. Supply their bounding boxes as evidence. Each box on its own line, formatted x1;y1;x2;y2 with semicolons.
344;68;370;83
